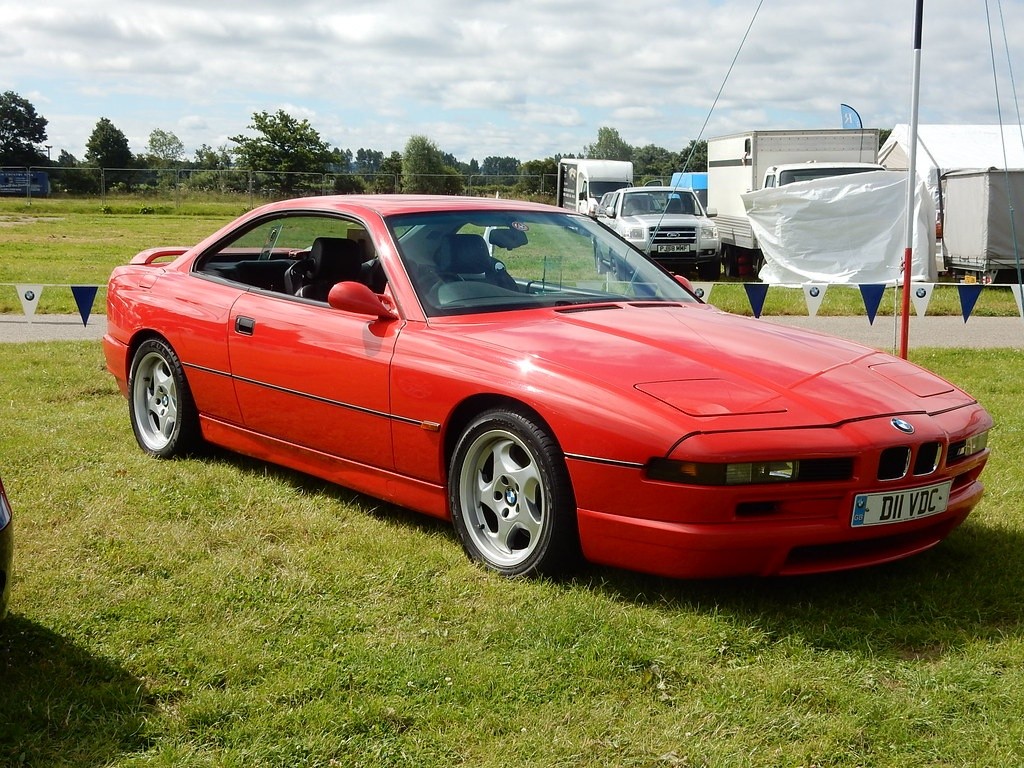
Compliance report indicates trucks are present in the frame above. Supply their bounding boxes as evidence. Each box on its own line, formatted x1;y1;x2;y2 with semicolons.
940;168;1024;291
706;129;887;277
557;158;635;236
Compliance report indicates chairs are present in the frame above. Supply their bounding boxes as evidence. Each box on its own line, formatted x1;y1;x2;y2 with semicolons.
664;198;685;213
624;199;644;215
292;234;518;303
232;261;290;295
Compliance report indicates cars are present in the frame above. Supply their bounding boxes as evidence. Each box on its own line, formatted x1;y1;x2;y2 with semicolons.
103;194;992;579
592;186;722;283
